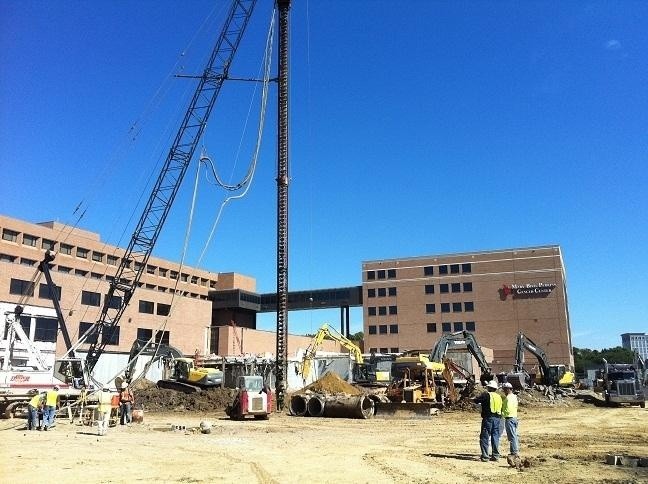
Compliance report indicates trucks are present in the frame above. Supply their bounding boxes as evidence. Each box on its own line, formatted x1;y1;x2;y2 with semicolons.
603;359;645;408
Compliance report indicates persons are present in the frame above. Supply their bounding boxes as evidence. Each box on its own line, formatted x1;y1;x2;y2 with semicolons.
469;380;503;462
499;382;519;456
27;392;47;430
39;385;60;431
95;384;114;436
118;382;134;425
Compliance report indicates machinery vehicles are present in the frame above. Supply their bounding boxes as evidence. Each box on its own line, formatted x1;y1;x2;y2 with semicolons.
507;333;575;391
0;249;99;418
390;328;499;403
226;375;273;419
124;338;225;394
302;322;397;387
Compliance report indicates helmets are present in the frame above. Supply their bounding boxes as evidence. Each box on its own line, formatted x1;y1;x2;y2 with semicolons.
502;382;513;388
486;380;498;389
121;382;128;389
103;384;109;389
54;385;59;390
